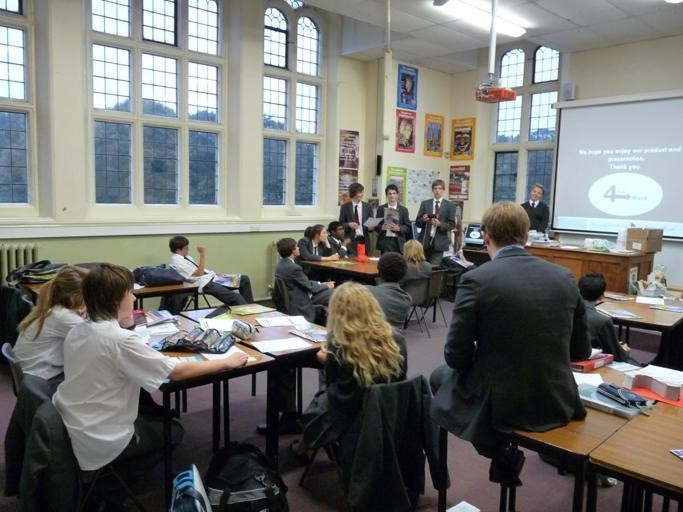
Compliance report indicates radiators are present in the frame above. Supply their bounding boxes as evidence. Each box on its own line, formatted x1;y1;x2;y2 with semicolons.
1;242;40;287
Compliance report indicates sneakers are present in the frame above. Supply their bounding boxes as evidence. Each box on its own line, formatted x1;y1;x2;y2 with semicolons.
488;441;524;484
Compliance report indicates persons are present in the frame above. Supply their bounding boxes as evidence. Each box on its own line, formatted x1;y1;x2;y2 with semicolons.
430;201;593;486
300;281;406;448
169;237;253;305
11;266;84;380
51;265;247;511
397;118;413;148
349;150;359;178
521;184;549;234
400;74;414;105
277;180;474;323
454;131;470;154
578;271;631;362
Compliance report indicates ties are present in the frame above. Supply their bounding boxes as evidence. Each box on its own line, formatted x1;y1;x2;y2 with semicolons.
531;203;536;209
355;205;360;225
435;201;442;216
184;256;207;274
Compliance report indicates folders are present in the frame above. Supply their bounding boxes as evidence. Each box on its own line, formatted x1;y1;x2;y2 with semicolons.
570;353;613;373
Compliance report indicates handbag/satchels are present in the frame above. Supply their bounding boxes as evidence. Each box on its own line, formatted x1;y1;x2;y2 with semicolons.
171;464;211;511
135;265;185;286
205;442;290;512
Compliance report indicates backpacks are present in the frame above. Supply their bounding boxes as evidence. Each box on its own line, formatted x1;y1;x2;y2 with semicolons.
6;260;67;285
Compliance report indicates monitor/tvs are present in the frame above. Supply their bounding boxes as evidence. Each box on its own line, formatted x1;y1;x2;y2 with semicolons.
463;223;485;246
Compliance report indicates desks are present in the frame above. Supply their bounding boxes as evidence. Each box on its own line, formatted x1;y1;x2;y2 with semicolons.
594;291;682;370
435;359;683;511
23;278;200;311
519;238;656;294
146;302;326;511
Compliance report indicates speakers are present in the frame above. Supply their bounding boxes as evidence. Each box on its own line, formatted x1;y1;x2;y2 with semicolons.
561;80;575;100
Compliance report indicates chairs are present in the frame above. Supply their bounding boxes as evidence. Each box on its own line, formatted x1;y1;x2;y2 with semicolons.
267;229;452;340
296;373;428;511
2;340;145;511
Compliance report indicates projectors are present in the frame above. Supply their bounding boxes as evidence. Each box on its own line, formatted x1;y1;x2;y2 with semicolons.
475;87;517;104
